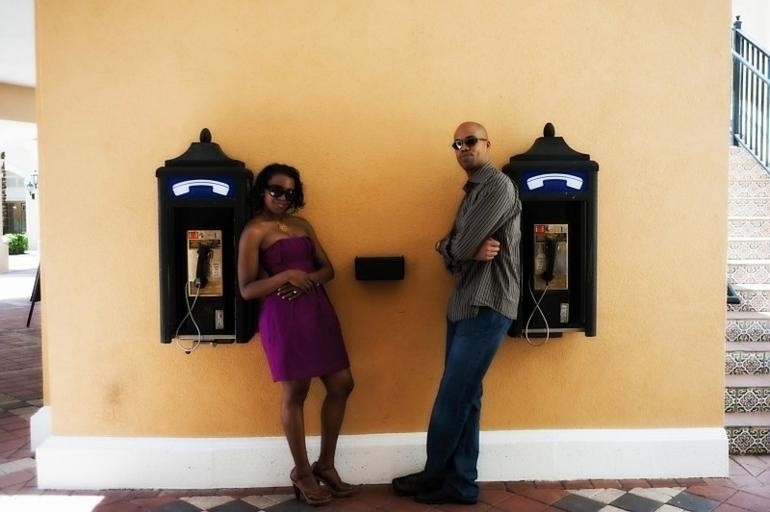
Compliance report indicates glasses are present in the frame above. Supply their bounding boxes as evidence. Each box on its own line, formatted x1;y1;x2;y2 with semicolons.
264;184;295;202
451;137;488;150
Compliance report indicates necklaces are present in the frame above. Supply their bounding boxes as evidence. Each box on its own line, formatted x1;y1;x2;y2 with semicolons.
277;220;288;232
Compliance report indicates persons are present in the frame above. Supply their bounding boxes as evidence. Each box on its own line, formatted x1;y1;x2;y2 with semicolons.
236;161;361;505
389;120;523;506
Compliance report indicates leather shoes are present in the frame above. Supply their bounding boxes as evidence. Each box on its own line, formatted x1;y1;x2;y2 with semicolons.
392;471;441;493
414;488;476;505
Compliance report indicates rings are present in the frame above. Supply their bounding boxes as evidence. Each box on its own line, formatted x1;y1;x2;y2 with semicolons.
293;290;297;294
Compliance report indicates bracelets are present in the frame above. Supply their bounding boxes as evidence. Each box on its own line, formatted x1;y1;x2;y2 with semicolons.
434;239;443;253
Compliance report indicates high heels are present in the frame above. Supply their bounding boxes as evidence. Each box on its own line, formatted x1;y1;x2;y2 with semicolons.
290;466;333;505
311;462;355;495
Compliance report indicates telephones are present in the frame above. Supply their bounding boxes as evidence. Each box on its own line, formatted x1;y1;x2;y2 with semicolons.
194;245;210;290
541;238;558;282
